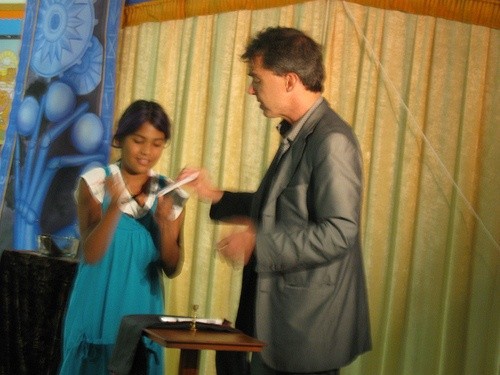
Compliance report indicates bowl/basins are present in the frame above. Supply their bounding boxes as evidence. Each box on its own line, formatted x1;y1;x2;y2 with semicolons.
37;235;79;259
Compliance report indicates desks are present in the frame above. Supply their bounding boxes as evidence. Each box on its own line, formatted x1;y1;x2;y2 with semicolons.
125;315;265;375
0;249;81;375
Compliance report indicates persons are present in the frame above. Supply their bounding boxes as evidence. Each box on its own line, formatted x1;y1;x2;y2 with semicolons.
59;99;190;375
177;24;374;375
